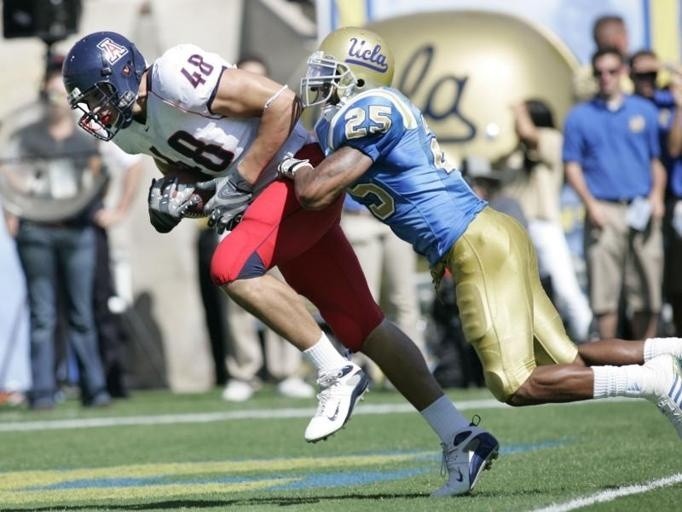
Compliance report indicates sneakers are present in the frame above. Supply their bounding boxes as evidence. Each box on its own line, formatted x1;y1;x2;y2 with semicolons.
431;421;501;499
221;376;315;403
303;361;370;444
0;381;113;413
641;352;681;439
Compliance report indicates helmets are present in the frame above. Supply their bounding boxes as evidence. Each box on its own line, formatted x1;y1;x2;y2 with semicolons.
298;26;396;109
62;32;147;142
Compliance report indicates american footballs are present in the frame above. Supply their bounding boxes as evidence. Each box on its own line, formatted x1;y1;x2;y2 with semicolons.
149;171;214;219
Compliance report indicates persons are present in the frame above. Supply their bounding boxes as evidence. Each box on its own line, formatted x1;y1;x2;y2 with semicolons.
63;30;500;497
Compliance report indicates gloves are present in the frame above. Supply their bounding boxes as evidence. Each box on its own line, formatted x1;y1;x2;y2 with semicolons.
277;154;314;180
147;175;195;234
196;168;257;236
